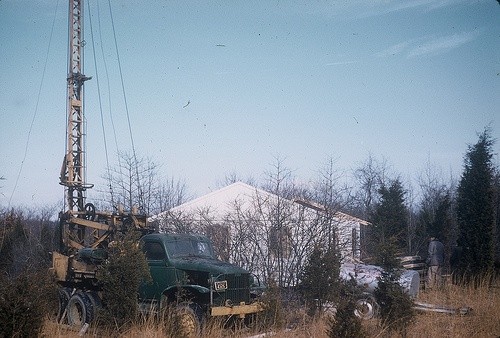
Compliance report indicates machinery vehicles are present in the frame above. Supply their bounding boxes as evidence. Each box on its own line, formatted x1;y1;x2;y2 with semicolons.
44;1;269;338
